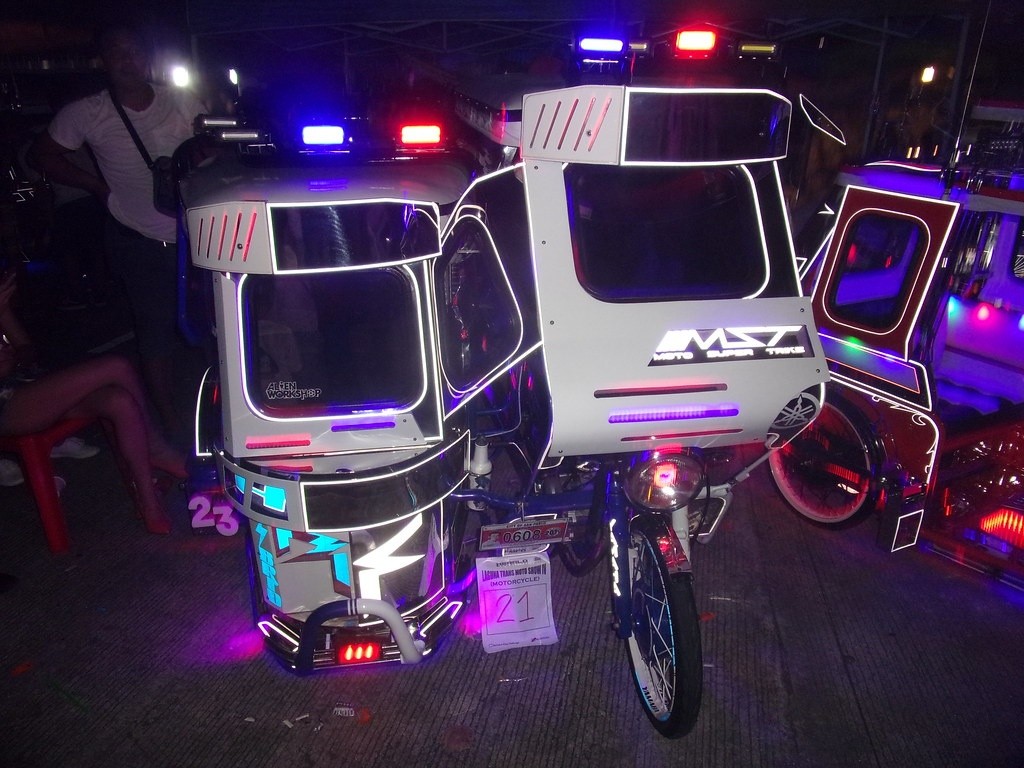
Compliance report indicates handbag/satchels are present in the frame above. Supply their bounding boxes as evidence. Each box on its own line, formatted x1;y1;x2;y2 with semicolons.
152;155;182;220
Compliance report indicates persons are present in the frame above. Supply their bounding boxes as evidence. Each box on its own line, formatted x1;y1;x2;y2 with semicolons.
0;267;190;535
25;19;211;465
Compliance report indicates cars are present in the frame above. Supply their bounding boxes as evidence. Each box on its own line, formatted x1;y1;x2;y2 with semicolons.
771;160;1024;607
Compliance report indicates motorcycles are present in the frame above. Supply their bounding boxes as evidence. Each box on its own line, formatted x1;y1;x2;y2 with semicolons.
174;15;846;740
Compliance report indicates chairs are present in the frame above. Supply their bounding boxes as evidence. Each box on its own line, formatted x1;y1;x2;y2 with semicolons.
0;413;101;557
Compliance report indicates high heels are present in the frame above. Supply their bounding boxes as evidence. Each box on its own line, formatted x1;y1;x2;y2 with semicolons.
138;493;172;535
150;453;191;479
